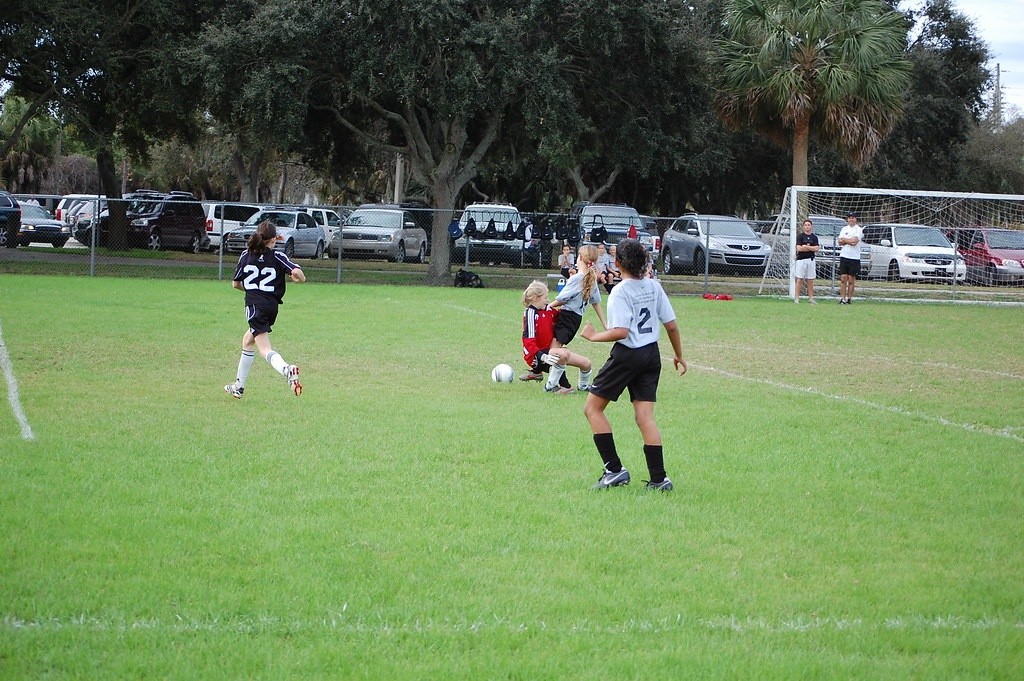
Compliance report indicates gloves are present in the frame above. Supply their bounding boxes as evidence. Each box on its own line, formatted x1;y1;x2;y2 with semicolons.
541;353;560;365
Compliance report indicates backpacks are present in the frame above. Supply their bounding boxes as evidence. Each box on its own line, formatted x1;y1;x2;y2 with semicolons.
449;211;582;242
627;216;636;238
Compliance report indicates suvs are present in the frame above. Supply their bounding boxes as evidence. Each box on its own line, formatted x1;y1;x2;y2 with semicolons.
661;212;771;276
98;189;208;254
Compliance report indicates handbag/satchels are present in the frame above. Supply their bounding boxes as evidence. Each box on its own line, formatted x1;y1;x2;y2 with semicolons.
590;215;608;242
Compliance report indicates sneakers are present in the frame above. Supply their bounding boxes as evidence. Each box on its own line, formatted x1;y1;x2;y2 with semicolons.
846;299;851;304
641;477;673;492
224;385;245;399
519;371;543;380
795;299;798;304
284;365;302;397
577;384;597;391
808;299;816;304
559;386;572;393
839;299;845;304
591;462;630;489
545;384;561;392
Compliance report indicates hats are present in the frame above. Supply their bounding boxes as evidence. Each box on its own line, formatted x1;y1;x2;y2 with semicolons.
848;212;855;217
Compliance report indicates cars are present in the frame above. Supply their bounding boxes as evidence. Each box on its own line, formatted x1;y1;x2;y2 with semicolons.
945;227;1024;287
859;223;966;285
760;213;872;279
0;190;661;269
331;210;427;264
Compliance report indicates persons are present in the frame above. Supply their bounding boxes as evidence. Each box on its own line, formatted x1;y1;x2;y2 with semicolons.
580;238;688;495
520;246;609;394
794;220;819;305
521;280;592;392
224;221;306;401
558;246;652;294
838;212;862;305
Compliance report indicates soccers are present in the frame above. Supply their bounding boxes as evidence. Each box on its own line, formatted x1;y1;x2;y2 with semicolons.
492;364;515;382
